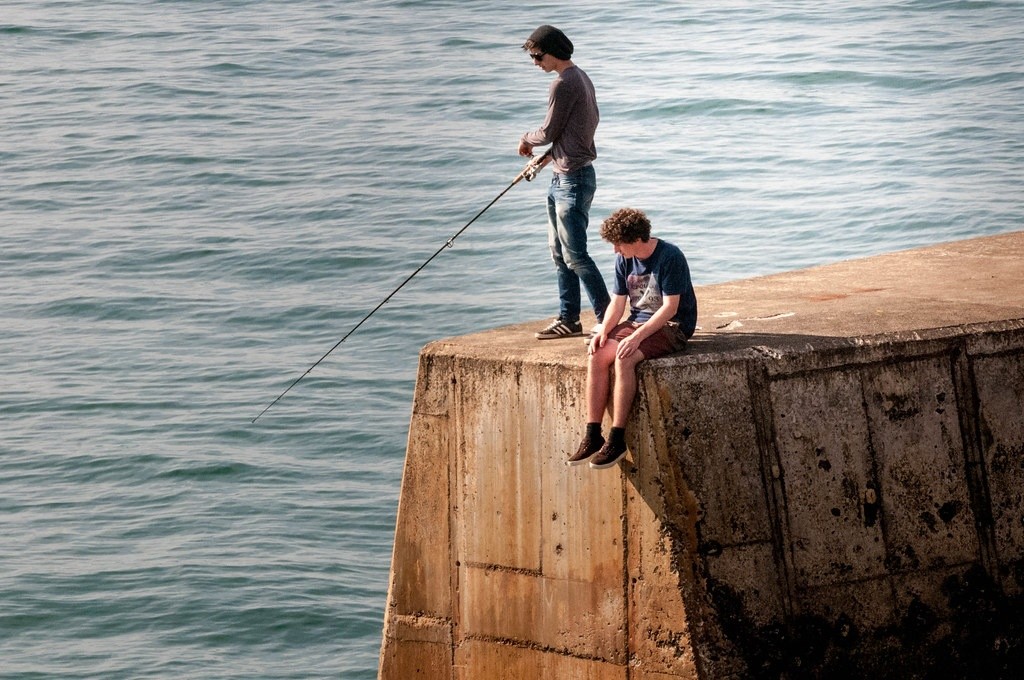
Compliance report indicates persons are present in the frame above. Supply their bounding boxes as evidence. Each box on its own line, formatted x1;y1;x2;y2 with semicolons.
567;208;698;469
519;26;611;340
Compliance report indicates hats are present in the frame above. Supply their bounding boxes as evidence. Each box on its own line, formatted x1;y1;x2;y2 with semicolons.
527;25;573;60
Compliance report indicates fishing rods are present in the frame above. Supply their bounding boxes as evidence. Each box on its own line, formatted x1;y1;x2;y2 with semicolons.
251;146;553;424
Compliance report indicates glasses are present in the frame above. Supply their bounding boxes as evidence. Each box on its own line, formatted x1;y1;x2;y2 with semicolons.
530;52;547;61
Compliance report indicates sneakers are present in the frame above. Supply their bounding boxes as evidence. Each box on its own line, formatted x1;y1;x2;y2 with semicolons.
565;436;606;466
535;318;582;339
589;442;629;469
584;332;597;344
